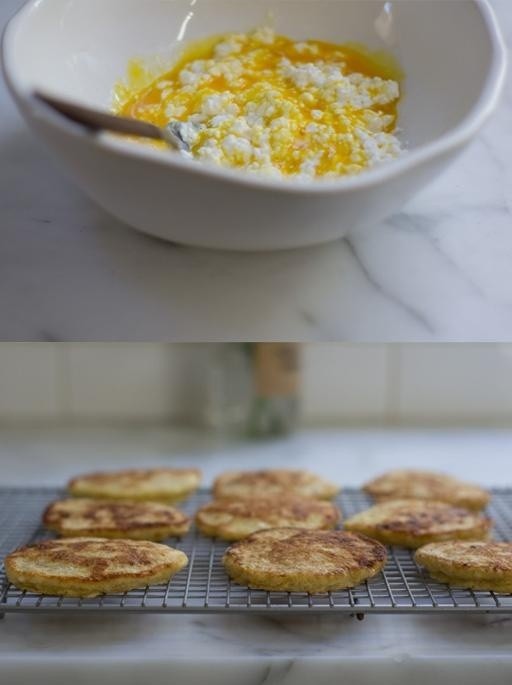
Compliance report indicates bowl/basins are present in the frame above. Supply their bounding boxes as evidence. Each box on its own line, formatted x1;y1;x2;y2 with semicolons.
0;0;508;255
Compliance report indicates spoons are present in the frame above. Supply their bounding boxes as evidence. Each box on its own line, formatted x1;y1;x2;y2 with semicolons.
31;89;201;151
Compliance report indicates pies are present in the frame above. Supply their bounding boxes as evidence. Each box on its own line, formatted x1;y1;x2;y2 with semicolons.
5;466;512;596
